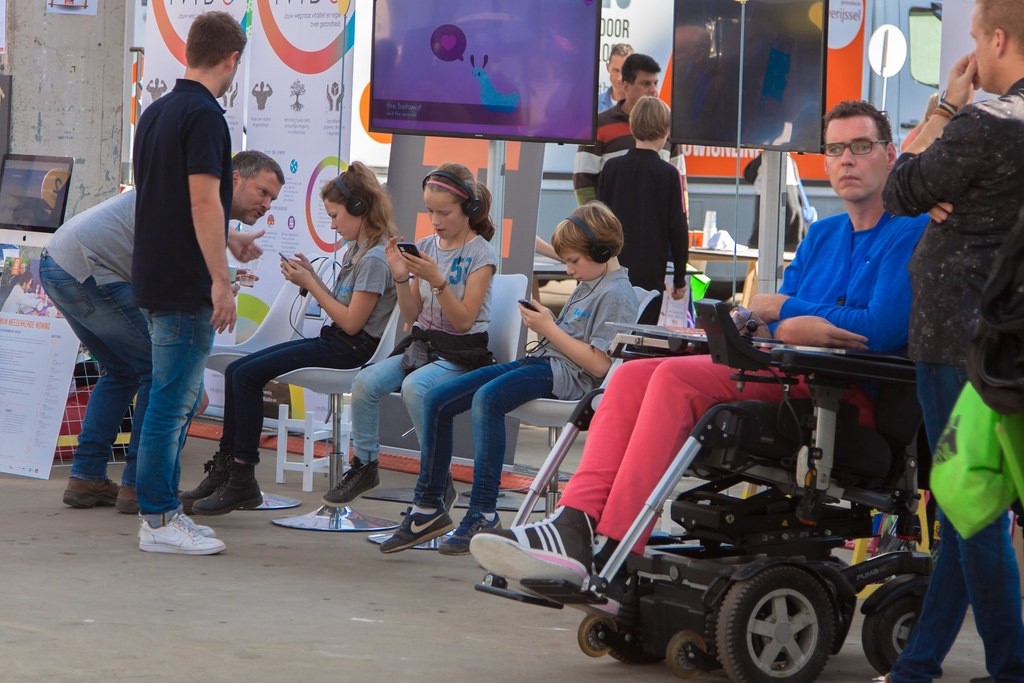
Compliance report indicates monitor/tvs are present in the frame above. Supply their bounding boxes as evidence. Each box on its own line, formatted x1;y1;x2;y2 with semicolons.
0;152;76;259
668;0;829;154
368;0;602;146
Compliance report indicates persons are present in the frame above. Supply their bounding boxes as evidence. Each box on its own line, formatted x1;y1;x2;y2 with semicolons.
596;96;689;325
131;11;248;556
900;92;938;154
881;0;1024;683
535;44;816;264
37;148;285;514
673;20;783;141
467;99;931;611
0;262;49;314
321;162;499;512
175;160;397;513
380;201;640;554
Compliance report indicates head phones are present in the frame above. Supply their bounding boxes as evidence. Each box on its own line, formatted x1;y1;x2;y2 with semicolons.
565;216;612;263
333;177;367;216
421;170;482;218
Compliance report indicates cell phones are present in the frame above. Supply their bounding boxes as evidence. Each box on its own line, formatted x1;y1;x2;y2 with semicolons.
278;252;288;262
517;299;539;312
397;242;421;258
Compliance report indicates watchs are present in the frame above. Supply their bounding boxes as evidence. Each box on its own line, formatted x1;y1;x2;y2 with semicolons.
432;279;447;295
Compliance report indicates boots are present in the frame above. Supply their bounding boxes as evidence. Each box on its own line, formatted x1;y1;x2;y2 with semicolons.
192;457;263;516
180;452;228;515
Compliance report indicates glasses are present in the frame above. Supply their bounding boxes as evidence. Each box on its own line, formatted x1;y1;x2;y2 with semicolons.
821;138;890;156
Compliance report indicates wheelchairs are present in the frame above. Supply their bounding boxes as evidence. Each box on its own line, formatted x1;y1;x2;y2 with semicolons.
474;295;933;683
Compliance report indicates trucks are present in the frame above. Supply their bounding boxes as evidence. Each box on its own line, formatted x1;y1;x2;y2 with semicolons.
356;0;942;280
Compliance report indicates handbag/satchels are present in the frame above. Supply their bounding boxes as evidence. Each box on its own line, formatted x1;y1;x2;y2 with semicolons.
929;381;1024;540
968;206;1024;414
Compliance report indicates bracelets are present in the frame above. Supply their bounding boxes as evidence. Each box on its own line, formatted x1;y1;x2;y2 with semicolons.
936;101;957;118
393;275;414;283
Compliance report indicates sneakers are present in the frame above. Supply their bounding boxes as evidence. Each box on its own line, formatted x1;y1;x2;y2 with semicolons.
469;507;595;586
63;477;120;507
520;531;629;594
116;486;139;514
379;499;456;553
443;475;459;512
322;456;380;507
439;511;502;554
138;513;216;537
139;517;226;555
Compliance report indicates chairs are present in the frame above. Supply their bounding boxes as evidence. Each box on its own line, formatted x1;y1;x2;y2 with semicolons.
203;250;660;550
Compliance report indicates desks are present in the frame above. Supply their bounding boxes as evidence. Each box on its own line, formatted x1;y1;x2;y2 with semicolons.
687;248;798;308
